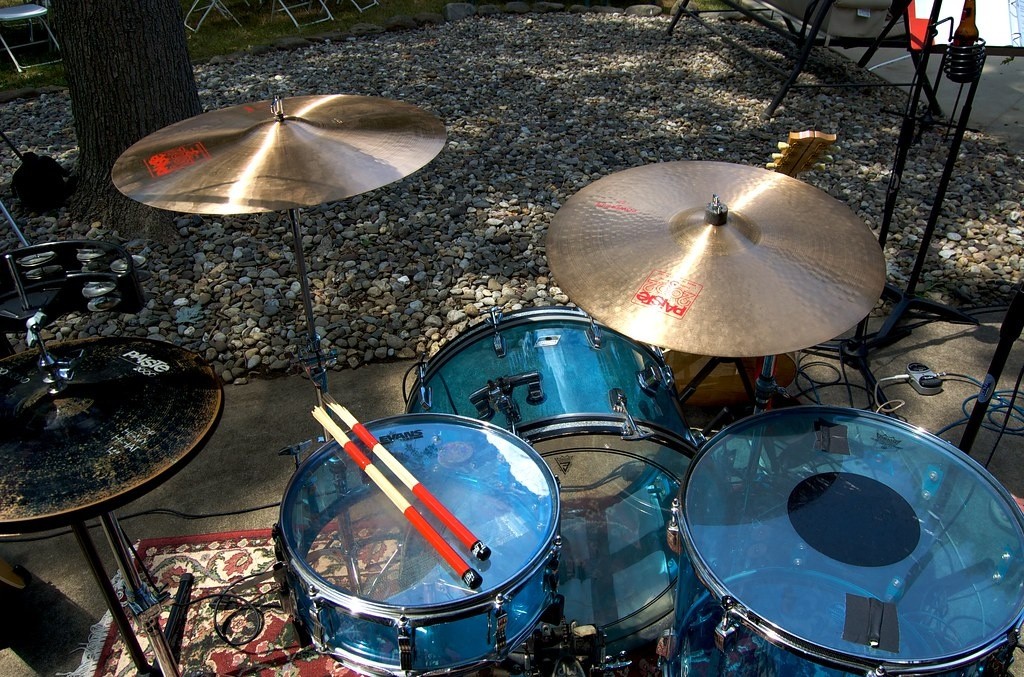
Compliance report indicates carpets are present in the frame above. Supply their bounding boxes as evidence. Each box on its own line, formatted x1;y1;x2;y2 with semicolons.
57;528;362;677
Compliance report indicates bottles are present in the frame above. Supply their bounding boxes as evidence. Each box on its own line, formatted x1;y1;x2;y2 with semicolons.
950;0;979;70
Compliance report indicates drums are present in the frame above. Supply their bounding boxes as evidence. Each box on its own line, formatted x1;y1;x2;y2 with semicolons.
657;404;1024;677
400;307;697;647
273;410;561;677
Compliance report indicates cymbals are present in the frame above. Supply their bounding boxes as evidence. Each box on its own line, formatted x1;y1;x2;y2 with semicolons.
0;241;147;349
110;95;448;218
0;336;225;531
546;158;888;360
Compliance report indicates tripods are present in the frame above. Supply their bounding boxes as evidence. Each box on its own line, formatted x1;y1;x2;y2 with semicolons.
794;0;987;420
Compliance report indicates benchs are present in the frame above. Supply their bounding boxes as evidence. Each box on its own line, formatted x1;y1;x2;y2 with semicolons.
667;0;946;120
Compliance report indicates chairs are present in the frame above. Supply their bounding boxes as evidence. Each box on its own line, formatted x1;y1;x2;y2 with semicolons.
0;0;63;72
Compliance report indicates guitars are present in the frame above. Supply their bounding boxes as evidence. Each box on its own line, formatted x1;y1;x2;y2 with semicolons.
661;129;837;414
